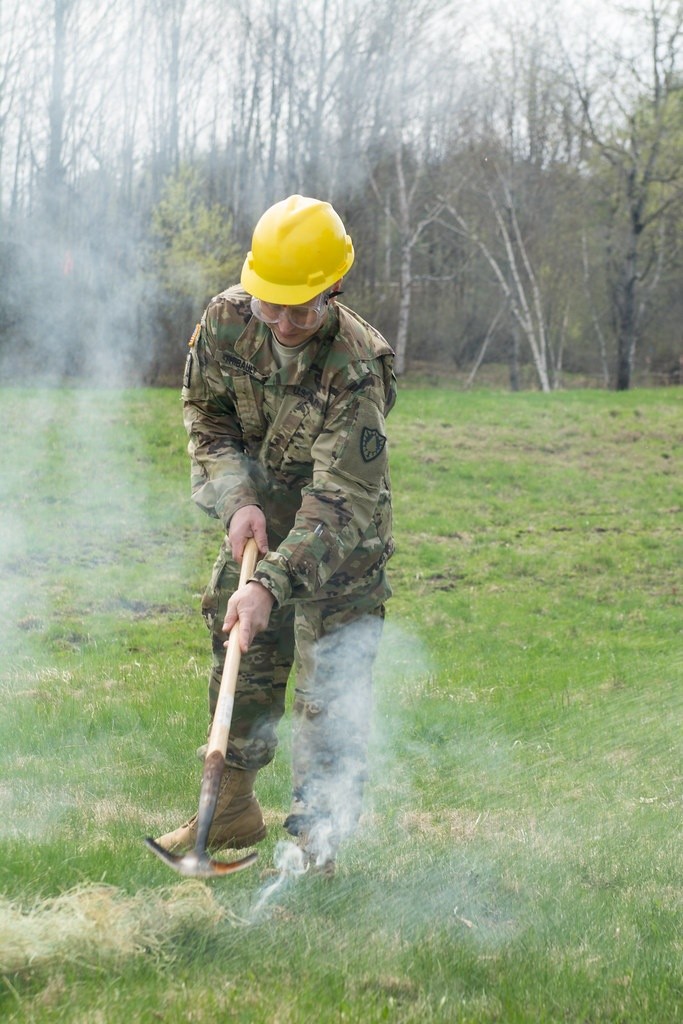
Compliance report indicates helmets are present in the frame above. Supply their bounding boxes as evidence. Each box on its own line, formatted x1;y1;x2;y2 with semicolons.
240;194;356;306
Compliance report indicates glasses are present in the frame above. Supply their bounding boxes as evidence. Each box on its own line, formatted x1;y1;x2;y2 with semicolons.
250;291;328;330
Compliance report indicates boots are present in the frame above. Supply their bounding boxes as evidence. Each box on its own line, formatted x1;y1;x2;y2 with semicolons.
284;830;334;881
153;764;267;858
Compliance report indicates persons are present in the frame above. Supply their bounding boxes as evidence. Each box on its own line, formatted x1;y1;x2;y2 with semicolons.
144;194;398;856
